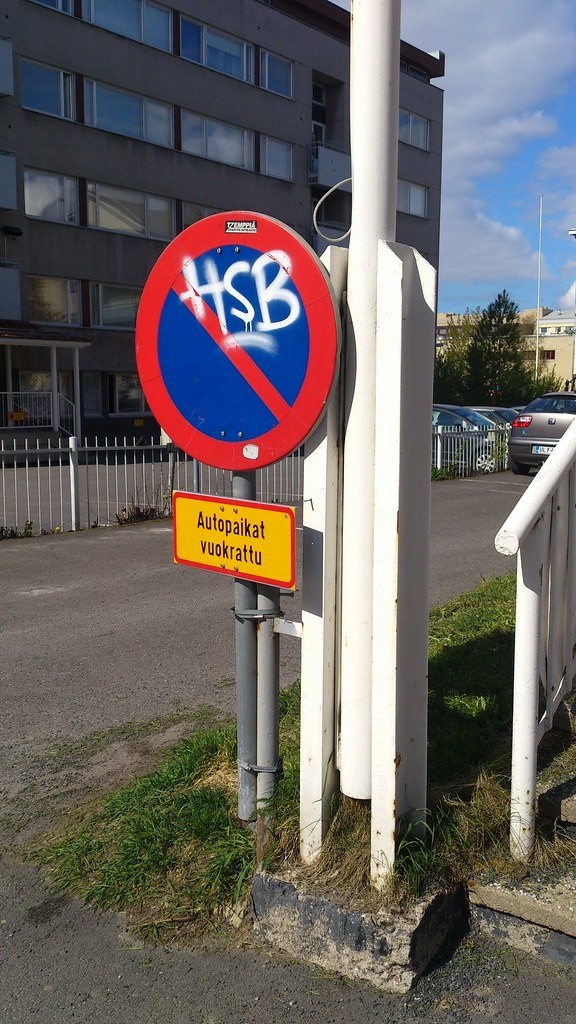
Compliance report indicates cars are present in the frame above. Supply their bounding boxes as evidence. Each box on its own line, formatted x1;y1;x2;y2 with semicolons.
506;390;576;475
432;402;527;473
